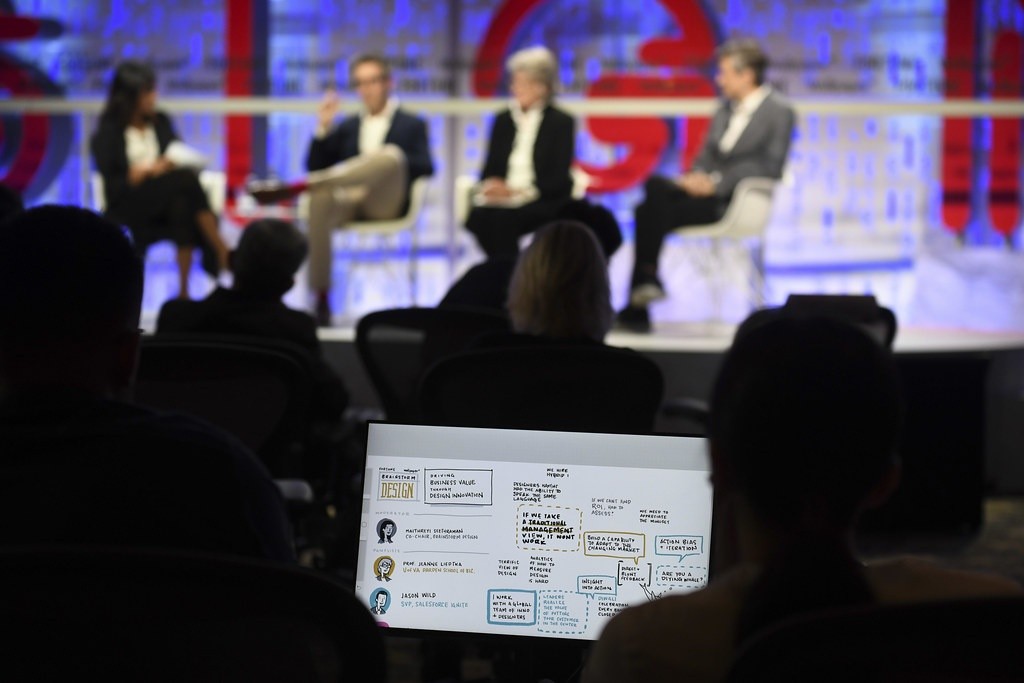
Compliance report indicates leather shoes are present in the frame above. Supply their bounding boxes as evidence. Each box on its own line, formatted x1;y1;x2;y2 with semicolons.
249;178;291;210
614;274;663;333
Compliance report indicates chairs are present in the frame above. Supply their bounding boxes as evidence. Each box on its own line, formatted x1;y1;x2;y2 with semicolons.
3;150;1024;683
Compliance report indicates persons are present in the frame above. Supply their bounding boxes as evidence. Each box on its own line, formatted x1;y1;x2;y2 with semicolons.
245;53;435;326
572;296;1024;683
420;195;624;359
2;204;320;683
606;35;797;333
158;215;347;406
88;59;237;301
403;221;667;435
460;42;576;262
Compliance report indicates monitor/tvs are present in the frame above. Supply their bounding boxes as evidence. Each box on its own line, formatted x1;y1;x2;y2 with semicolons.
348;418;723;648
